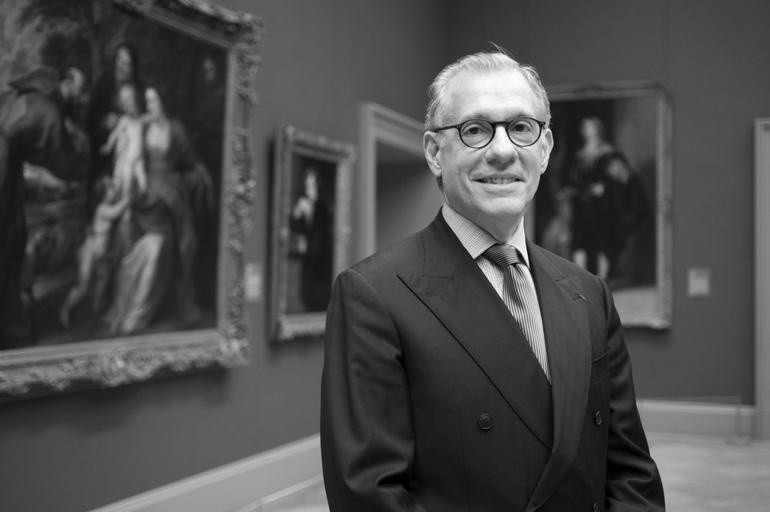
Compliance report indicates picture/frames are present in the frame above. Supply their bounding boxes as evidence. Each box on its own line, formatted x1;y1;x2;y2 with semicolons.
267;120;358;344
0;1;264;396
522;76;678;332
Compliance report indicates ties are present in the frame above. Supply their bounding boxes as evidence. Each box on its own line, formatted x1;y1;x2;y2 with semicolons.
484;241;551;385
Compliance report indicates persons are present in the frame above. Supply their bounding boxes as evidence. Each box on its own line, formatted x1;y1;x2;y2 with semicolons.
563;114;631;282
289;167;334;311
318;41;667;512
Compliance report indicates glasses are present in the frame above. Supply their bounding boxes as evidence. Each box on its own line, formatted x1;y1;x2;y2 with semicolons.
432;114;548;148
